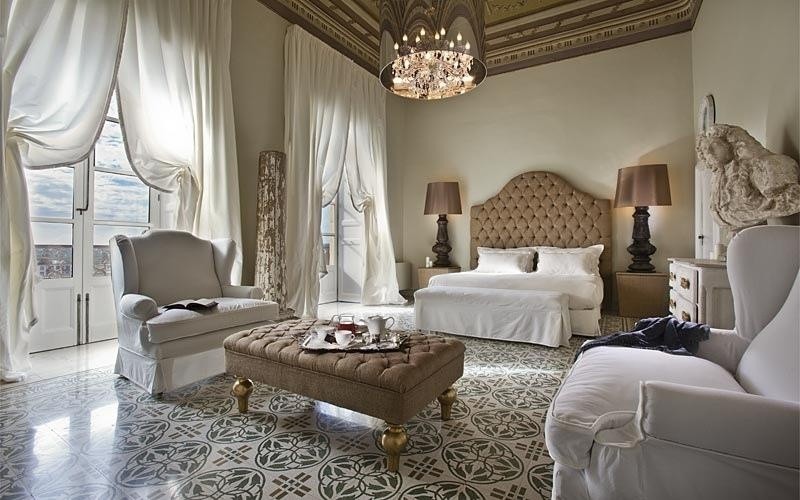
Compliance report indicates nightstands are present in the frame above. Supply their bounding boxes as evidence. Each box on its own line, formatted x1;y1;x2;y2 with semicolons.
417;266;460;289
614;270;670;316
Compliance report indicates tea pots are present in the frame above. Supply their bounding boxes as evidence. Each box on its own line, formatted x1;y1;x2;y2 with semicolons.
359;316;395;335
332;314;355;338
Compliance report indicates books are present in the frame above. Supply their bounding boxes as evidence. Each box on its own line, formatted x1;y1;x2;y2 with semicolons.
163;297;218;311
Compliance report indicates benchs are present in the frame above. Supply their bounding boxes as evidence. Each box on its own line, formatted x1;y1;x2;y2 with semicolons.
411;286;575;348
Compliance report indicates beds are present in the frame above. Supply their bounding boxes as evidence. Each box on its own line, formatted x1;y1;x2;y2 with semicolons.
427;169;614;337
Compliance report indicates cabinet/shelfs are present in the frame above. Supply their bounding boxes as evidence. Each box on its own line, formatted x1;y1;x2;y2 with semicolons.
666;257;736;329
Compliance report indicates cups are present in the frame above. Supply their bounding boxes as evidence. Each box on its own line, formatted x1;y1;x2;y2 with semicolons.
309;326;335;342
335;330;355;344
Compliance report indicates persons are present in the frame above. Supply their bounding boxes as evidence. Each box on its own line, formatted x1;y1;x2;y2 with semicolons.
693;124;799;261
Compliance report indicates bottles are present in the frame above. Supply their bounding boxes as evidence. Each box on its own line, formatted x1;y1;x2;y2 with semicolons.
430;261;433;267
426;257;430;268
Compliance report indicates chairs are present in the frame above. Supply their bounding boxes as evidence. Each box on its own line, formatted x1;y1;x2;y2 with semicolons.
543;222;799;499
108;229;282;398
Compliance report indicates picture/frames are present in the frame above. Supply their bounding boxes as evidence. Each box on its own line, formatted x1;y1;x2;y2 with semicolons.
698;95;715;136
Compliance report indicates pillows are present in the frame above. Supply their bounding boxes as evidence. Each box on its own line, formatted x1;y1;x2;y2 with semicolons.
474;244;604;276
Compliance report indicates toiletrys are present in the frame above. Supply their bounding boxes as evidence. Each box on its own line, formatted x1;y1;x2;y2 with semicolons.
425;256;433;268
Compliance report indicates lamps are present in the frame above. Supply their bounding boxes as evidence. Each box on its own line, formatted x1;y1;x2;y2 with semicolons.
612;163;673;273
423;181;463;267
375;0;493;100
